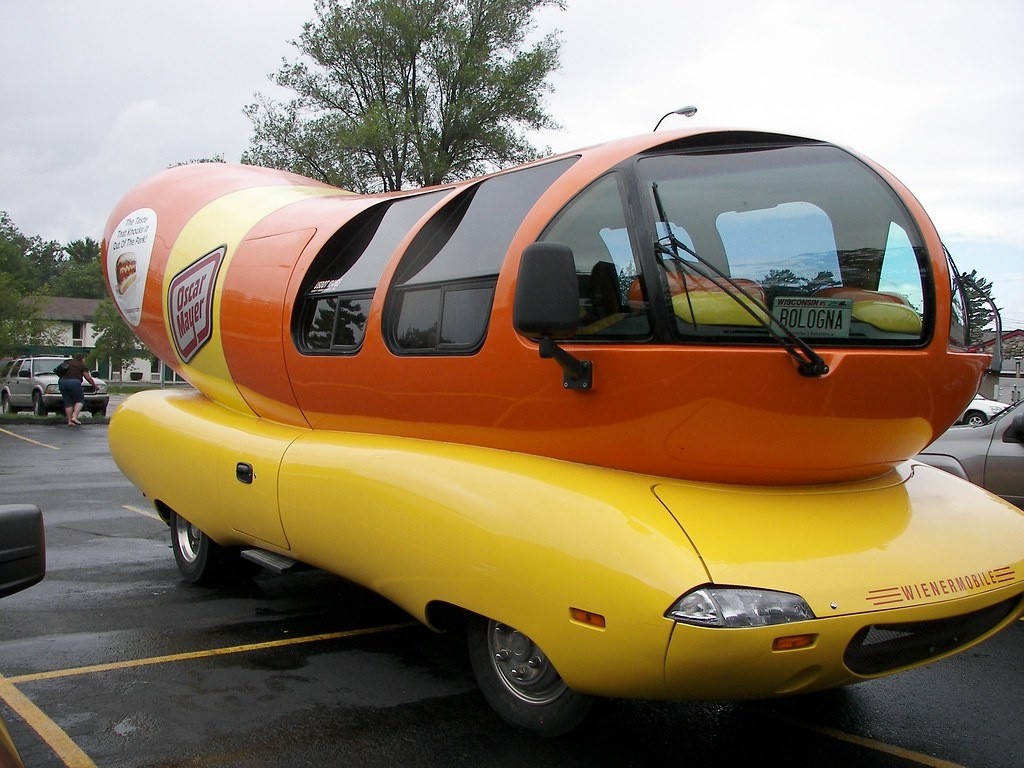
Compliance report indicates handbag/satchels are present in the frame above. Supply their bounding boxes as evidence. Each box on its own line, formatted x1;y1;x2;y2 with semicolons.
53;360;69;377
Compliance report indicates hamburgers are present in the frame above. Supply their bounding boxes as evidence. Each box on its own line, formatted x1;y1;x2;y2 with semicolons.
115;252;137;294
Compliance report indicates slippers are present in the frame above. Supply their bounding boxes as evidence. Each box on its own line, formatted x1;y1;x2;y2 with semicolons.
71;419;81;425
67;424;78;427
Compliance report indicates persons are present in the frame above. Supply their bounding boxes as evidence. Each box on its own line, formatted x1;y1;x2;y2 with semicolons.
52;353;99;427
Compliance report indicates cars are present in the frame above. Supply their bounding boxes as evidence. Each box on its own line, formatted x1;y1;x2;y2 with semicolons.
1;357;110;420
909;397;1024;510
954;393;1014;427
